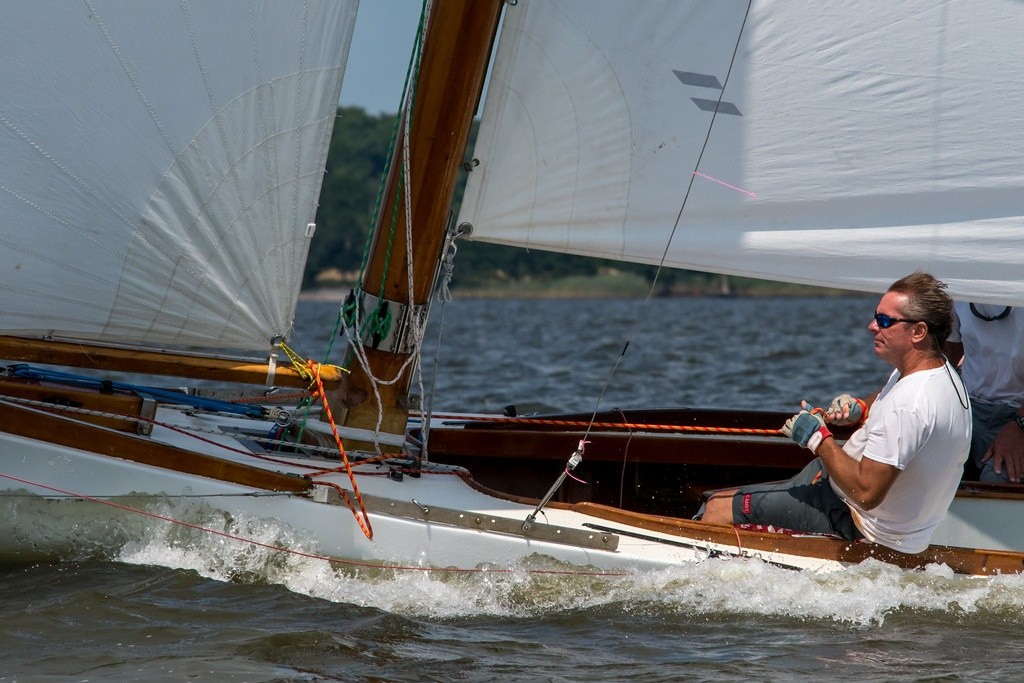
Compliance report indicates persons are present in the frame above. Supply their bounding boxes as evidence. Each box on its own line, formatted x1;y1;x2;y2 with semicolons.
701;272;973;556
947;300;1024;484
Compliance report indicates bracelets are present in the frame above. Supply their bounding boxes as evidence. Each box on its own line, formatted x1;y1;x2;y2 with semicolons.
1010;413;1024;434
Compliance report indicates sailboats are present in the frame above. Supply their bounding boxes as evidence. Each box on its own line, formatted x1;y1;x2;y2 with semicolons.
0;0;1024;577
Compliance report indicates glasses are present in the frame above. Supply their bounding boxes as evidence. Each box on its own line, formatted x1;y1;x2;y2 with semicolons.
875;311;922;328
